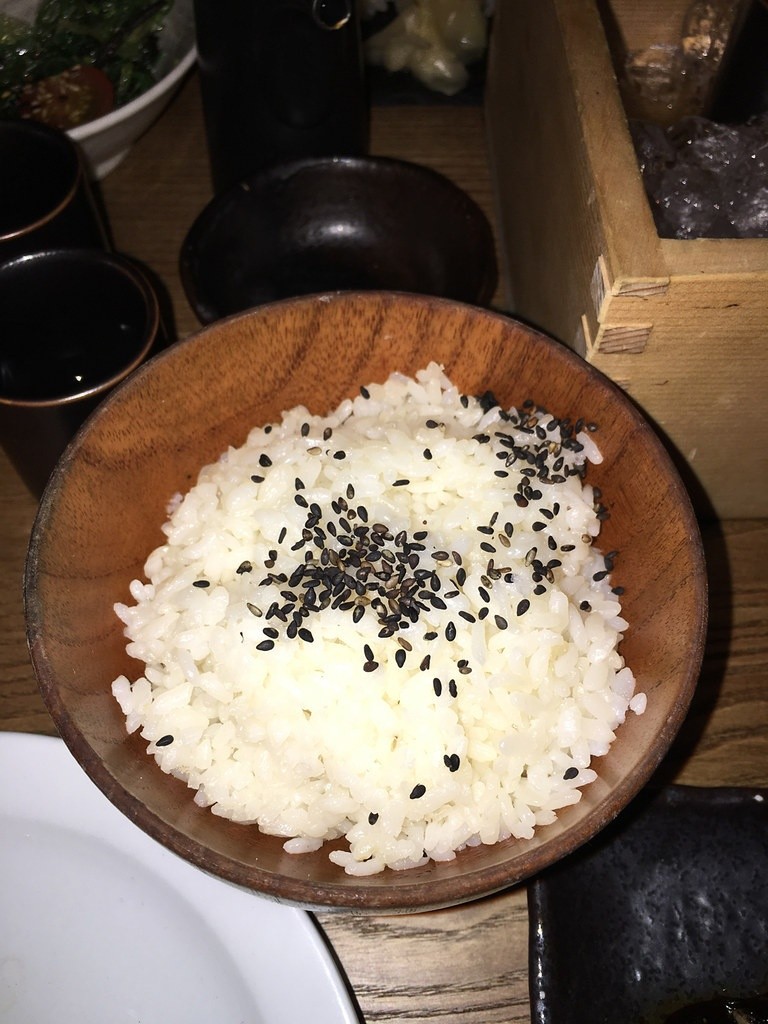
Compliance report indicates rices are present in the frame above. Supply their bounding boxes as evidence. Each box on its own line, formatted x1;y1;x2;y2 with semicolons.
111;362;645;878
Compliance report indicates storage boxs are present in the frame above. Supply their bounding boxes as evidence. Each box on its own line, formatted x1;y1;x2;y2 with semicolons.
492;0;767;532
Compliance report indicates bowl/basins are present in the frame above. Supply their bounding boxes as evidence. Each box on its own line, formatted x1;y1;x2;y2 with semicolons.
176;156;498;328
65;42;197;180
22;288;708;908
0;124;160;407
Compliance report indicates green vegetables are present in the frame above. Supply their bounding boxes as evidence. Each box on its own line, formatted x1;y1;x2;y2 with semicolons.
0;0;176;125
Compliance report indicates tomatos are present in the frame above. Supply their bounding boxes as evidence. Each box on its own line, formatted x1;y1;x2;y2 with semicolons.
17;64;114;131
